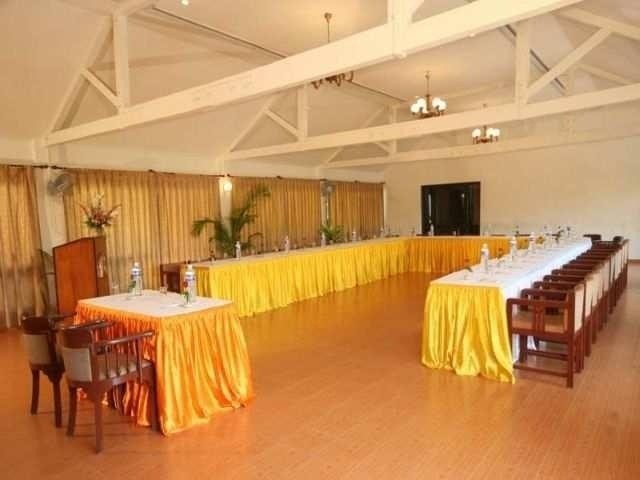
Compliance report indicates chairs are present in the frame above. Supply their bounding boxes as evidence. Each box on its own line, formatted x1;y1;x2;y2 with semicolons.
160;261;186;293
21;311;105;427
60;321;156;453
507;241;630;388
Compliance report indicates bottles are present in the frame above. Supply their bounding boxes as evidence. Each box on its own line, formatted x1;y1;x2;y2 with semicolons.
283;236;289;254
352;229;356;241
527;231;536;253
508;236;518;260
236;241;241;260
379;225;384;237
544;225;553;248
320;232;325;248
479;242;491;276
130;262;142;296
430;224;434;237
184;265;197;304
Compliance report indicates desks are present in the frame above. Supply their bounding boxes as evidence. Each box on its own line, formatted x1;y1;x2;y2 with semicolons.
407;237;592;385
181;235;408;319
71;289;253;435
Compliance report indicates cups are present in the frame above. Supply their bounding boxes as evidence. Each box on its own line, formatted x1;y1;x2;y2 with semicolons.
160;283;167;296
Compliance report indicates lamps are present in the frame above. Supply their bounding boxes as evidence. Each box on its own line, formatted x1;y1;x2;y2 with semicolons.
411;72;445;119
312;13;354;89
472;104;499;143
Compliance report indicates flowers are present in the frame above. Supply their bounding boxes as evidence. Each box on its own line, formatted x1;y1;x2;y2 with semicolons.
77;191;121;230
